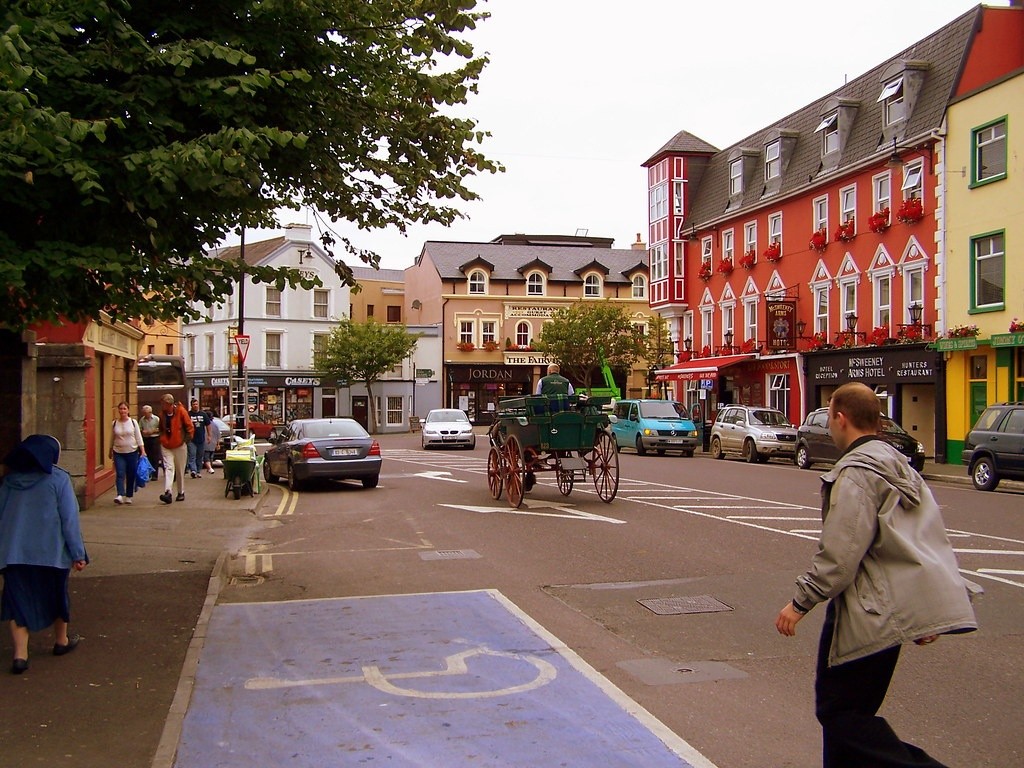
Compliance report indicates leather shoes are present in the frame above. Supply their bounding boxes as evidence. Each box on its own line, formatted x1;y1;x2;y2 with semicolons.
12;658;28;674
52;633;79;656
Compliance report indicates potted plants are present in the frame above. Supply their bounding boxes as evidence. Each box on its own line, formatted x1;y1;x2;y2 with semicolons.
484;342;500;351
456;342;474;351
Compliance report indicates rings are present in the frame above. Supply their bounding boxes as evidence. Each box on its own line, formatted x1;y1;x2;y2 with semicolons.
924;638;931;644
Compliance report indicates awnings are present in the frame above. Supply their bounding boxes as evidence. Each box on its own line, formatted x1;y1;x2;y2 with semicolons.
653;353;756;380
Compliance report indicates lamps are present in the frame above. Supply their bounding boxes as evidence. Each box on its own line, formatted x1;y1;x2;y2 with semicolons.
688;223;718;248
683;337;700;356
885;136;933;176
896;302;931;335
796;319;812;342
846;312;866;343
725;331;741;352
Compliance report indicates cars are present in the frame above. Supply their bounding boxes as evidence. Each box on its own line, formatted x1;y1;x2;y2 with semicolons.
262;416;383;492
418;409;476;450
190;413;279;463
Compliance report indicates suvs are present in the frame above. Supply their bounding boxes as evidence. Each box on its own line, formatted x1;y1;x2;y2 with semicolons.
961;402;1024;491
794;407;926;475
710;403;798;463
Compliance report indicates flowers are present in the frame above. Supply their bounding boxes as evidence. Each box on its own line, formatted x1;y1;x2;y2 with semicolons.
899;324;922;343
738;252;754;270
678;339;758;362
834;331;854;348
1008;319;1024;333
896;197;923;225
763;240;780;263
697;261;711;284
718;256;733;273
834;221;854;243
867;207;890;235
947;324;981;337
866;323;890;346
808;332;827;352
809;228;826;252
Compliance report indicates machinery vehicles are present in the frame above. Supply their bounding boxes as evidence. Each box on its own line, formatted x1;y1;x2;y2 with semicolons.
574;326;622;415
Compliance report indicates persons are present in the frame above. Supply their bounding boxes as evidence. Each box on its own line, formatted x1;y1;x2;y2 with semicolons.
673;404;688;418
108;401;147;505
138;404;160;480
0;433;90;675
158;393;195;504
579;391;588;400
535;364;574;410
204;411;221;474
187;398;211;478
775;382;979;768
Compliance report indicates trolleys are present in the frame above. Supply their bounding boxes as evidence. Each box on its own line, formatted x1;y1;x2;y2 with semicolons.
221;459;256;500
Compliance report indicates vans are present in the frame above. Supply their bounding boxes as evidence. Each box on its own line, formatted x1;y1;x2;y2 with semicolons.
610;399;703;457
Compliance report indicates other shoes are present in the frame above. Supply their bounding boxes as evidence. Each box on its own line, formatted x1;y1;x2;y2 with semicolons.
114;496;124;503
151;476;158;481
126;497;132;505
191;471;202;478
176;492;185;500
159;492;173;503
207;467;215;475
